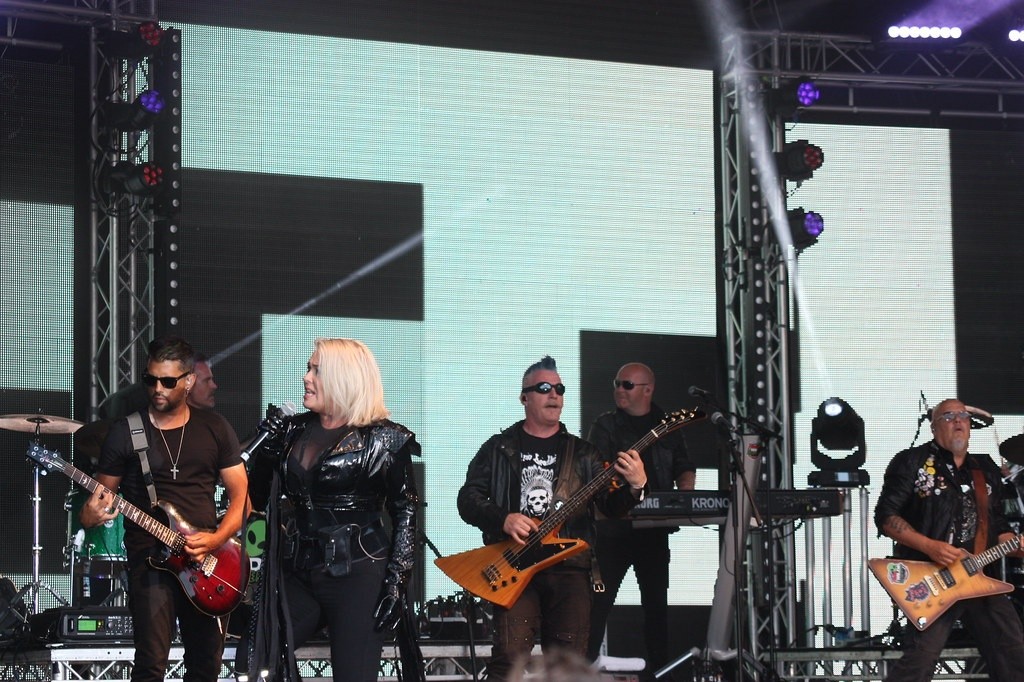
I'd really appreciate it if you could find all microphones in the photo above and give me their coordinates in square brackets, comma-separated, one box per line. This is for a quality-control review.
[240, 402, 296, 462]
[688, 386, 714, 397]
[922, 394, 929, 411]
[712, 411, 740, 434]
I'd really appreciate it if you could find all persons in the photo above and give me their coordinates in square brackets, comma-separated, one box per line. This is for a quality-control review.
[875, 398, 1024, 682]
[585, 363, 696, 682]
[457, 355, 650, 682]
[187, 353, 217, 410]
[80, 337, 248, 682]
[234, 338, 417, 682]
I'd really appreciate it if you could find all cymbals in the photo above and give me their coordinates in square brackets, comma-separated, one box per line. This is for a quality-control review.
[0, 413, 84, 435]
[927, 405, 995, 430]
[999, 433, 1024, 468]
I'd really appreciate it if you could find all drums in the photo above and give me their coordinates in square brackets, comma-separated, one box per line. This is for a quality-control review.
[63, 488, 129, 580]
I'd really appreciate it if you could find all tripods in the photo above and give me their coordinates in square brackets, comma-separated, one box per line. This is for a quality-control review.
[0, 421, 70, 629]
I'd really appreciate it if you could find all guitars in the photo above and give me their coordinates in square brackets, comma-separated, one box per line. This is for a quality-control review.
[867, 530, 1023, 633]
[432, 403, 709, 611]
[25, 439, 252, 620]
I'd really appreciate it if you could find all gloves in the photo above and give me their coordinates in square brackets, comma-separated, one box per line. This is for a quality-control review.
[373, 584, 409, 633]
[258, 408, 286, 448]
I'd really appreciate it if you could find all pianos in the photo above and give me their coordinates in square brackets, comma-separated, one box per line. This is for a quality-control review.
[592, 486, 845, 682]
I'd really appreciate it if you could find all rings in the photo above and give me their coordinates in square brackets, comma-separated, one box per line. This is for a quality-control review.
[105, 507, 110, 513]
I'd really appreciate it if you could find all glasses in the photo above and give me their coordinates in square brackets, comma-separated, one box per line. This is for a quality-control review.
[141, 370, 191, 389]
[932, 412, 970, 427]
[522, 382, 566, 396]
[613, 379, 648, 390]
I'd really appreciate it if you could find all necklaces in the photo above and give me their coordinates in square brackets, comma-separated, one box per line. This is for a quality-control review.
[154, 405, 186, 480]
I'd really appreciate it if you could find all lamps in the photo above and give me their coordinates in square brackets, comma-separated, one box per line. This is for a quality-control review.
[770, 76, 819, 118]
[125, 21, 165, 59]
[123, 163, 165, 197]
[128, 90, 164, 128]
[788, 206, 823, 254]
[871, 23, 966, 53]
[780, 137, 822, 185]
[808, 397, 871, 487]
